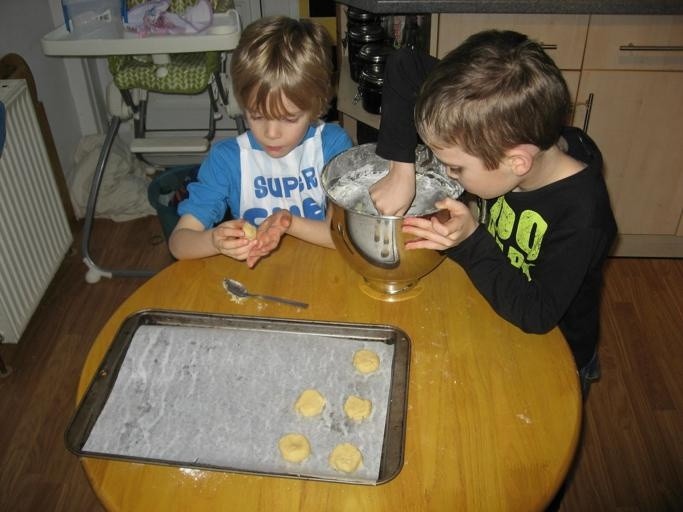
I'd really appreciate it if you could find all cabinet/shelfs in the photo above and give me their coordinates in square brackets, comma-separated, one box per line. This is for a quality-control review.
[333, 0, 683, 261]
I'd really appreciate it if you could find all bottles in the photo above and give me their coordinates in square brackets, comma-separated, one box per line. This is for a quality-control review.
[347, 7, 426, 114]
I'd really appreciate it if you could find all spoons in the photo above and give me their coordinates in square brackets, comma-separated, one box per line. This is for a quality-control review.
[222, 278, 309, 310]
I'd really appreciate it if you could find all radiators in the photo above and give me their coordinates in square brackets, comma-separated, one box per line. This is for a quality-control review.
[0, 79, 74, 378]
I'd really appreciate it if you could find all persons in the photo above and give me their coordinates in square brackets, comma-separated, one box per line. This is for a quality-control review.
[368, 28, 618, 406]
[168, 15, 357, 269]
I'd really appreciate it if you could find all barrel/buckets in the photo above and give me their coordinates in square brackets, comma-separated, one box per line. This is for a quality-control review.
[320, 141, 466, 301]
